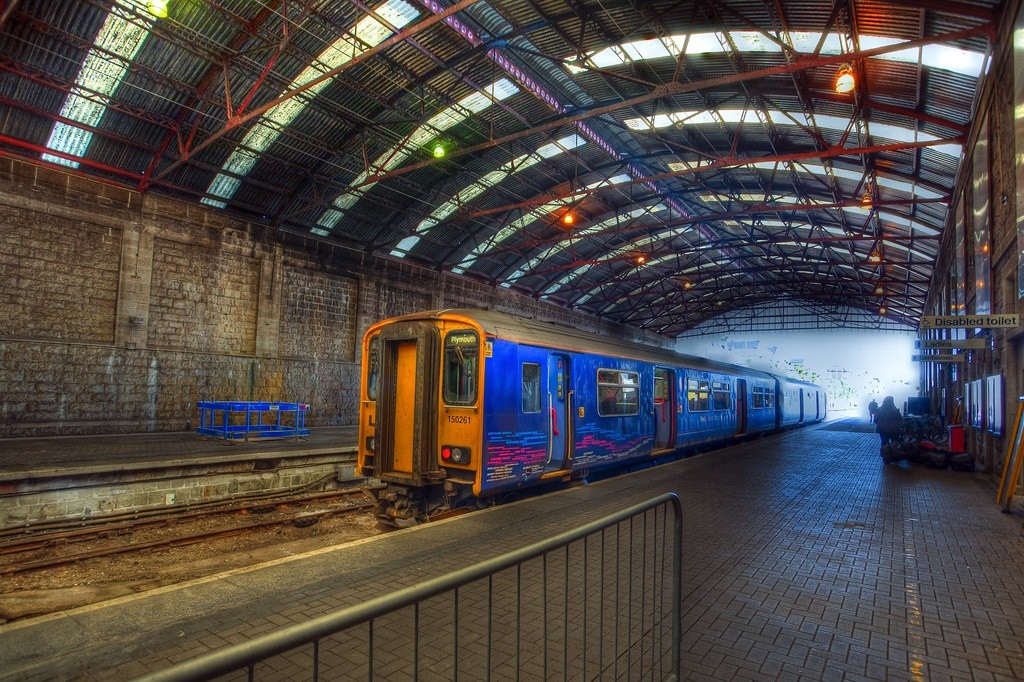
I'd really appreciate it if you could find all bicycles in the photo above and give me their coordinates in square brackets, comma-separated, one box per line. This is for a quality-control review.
[900, 413, 950, 444]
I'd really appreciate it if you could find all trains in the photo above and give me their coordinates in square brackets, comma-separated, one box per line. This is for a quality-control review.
[355, 310, 828, 527]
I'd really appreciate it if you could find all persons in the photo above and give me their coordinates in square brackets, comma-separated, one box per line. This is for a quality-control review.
[874, 396, 904, 445]
[868, 399, 878, 423]
